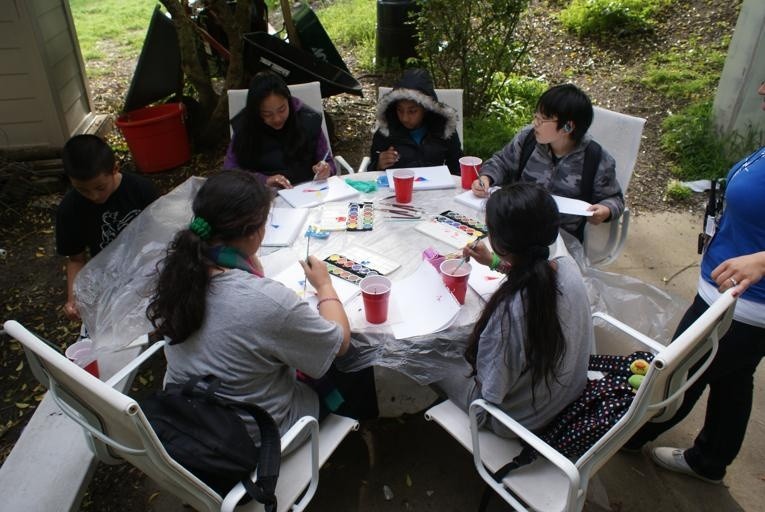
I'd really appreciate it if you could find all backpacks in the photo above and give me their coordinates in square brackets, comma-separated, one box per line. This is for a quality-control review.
[133, 373, 281, 512]
[477, 351, 654, 512]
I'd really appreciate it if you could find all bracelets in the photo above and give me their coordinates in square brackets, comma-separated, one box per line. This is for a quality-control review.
[316, 297, 342, 310]
[490, 252, 500, 271]
[501, 259, 511, 276]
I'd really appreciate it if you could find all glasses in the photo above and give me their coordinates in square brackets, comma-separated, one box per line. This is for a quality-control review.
[534, 115, 558, 126]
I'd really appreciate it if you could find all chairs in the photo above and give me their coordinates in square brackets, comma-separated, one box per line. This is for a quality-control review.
[358, 86, 464, 172]
[227, 80, 355, 175]
[4, 319, 359, 512]
[584, 106, 647, 268]
[424, 286, 742, 512]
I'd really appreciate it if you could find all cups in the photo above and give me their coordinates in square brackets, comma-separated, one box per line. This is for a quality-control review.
[359, 276, 391, 324]
[460, 156, 482, 189]
[440, 259, 471, 305]
[393, 171, 413, 202]
[66, 340, 99, 378]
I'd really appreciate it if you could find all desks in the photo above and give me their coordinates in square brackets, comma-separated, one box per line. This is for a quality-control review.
[255, 171, 566, 347]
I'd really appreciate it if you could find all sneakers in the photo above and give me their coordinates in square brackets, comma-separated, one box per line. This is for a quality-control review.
[621, 446, 640, 453]
[651, 446, 723, 486]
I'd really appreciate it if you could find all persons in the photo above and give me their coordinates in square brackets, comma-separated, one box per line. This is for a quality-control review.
[473, 83, 627, 235]
[424, 182, 593, 441]
[628, 72, 764, 485]
[223, 71, 335, 188]
[145, 173, 391, 512]
[369, 64, 464, 175]
[55, 134, 158, 318]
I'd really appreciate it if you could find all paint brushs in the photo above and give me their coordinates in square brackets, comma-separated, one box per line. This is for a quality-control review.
[384, 216, 420, 219]
[376, 151, 380, 153]
[313, 150, 329, 181]
[304, 236, 309, 286]
[474, 165, 483, 187]
[452, 238, 481, 274]
[384, 210, 408, 213]
[393, 212, 413, 216]
[399, 206, 417, 212]
[386, 203, 414, 208]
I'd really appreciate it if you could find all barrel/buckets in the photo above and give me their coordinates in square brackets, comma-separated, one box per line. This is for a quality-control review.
[114, 103, 192, 173]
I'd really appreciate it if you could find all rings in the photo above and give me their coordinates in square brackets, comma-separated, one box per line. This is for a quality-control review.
[325, 162, 328, 170]
[729, 277, 738, 285]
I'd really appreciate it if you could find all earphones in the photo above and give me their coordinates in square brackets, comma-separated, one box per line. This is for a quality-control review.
[564, 123, 571, 132]
